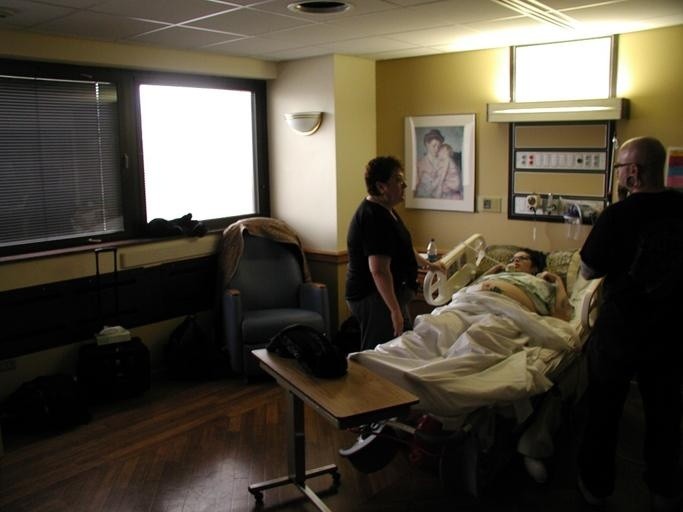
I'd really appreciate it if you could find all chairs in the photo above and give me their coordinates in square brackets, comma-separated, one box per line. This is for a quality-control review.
[216, 216, 331, 378]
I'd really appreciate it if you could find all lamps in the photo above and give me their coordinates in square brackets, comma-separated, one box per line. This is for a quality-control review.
[284, 111, 324, 136]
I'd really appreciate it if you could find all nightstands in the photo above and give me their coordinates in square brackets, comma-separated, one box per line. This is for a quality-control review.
[408, 246, 450, 325]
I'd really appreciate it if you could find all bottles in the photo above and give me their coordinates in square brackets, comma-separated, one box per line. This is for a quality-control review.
[427, 238, 437, 261]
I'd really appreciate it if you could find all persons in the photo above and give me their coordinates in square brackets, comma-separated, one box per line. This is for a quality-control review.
[415, 129, 462, 200]
[344, 157, 448, 351]
[580, 136, 683, 512]
[348, 248, 575, 395]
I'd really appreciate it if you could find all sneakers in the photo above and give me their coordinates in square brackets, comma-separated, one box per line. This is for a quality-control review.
[574, 467, 610, 501]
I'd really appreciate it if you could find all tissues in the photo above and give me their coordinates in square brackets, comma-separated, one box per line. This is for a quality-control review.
[95, 325, 132, 346]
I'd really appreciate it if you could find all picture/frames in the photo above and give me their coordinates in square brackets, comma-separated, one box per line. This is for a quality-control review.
[403, 113, 476, 214]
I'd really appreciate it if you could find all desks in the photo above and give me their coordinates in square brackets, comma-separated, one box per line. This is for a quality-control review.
[248, 346, 421, 512]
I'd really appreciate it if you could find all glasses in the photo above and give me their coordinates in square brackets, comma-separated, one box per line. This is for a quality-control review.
[612, 161, 633, 167]
[511, 255, 533, 261]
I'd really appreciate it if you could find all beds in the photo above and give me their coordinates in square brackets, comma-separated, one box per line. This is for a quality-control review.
[350, 230, 604, 512]
